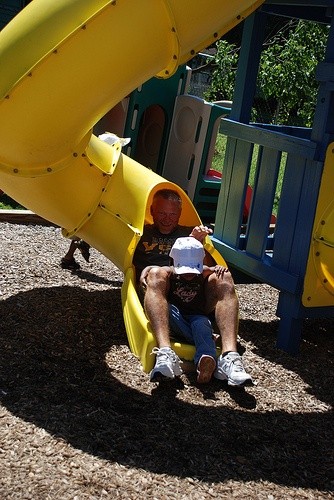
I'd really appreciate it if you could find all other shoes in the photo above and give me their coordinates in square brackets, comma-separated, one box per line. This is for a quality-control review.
[194, 355, 217, 384]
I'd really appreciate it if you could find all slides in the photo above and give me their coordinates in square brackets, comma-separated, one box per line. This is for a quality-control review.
[0, 1, 264, 373]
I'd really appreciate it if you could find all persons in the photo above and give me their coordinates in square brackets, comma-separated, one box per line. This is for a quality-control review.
[139, 236, 230, 384]
[61, 236, 91, 268]
[132, 188, 256, 387]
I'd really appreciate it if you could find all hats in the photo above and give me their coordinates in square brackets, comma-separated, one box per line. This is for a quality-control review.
[169, 236, 207, 277]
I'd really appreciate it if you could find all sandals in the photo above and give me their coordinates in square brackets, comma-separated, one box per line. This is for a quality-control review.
[74, 238, 91, 263]
[60, 255, 82, 269]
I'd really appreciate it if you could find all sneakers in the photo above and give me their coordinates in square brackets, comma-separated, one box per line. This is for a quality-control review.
[212, 353, 254, 389]
[146, 342, 183, 382]
